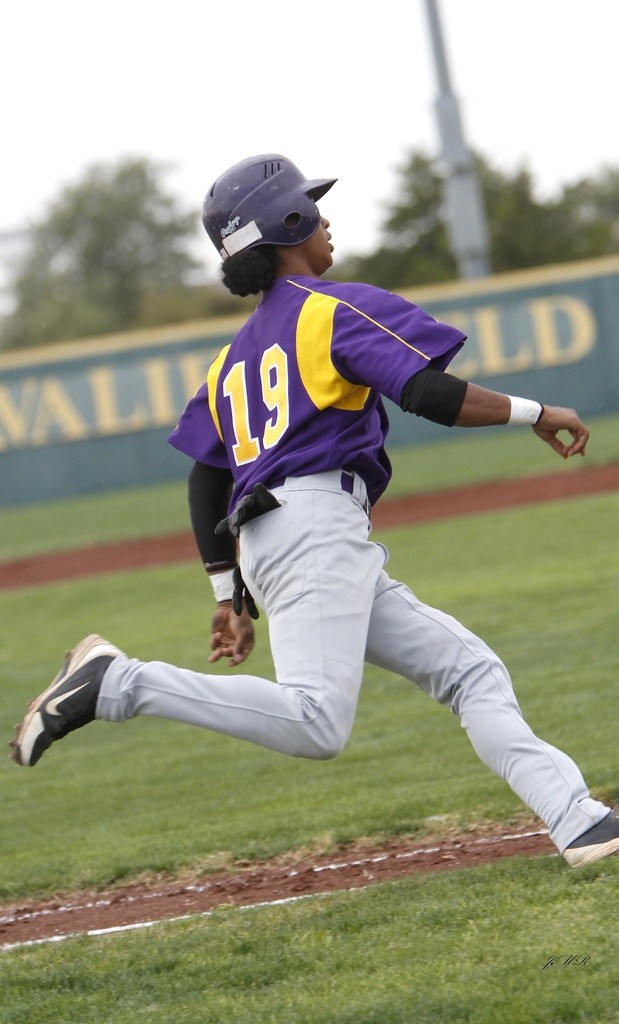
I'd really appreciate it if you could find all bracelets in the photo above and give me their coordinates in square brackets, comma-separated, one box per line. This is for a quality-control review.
[208, 566, 244, 606]
[504, 395, 544, 427]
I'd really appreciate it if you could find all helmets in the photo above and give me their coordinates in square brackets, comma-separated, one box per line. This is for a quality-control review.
[203, 154, 338, 260]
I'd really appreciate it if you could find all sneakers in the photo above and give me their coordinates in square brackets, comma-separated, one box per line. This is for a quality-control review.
[561, 809, 619, 870]
[8, 633, 122, 768]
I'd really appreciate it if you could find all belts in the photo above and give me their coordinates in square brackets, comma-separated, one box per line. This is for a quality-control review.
[268, 472, 373, 522]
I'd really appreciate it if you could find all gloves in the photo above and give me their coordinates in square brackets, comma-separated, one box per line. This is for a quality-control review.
[232, 564, 259, 619]
[214, 482, 281, 537]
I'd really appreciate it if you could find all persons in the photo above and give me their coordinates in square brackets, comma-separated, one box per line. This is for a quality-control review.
[7, 154, 619, 869]
